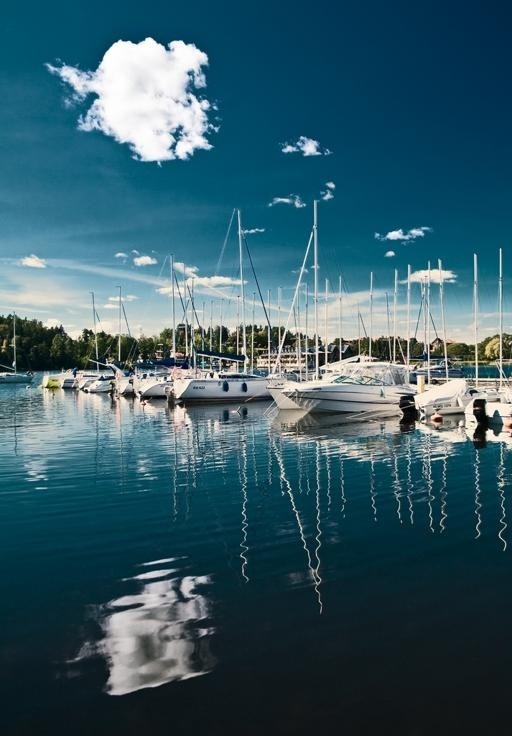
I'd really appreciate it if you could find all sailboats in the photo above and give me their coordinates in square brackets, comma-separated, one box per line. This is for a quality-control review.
[42, 199, 512, 441]
[0, 310, 36, 382]
